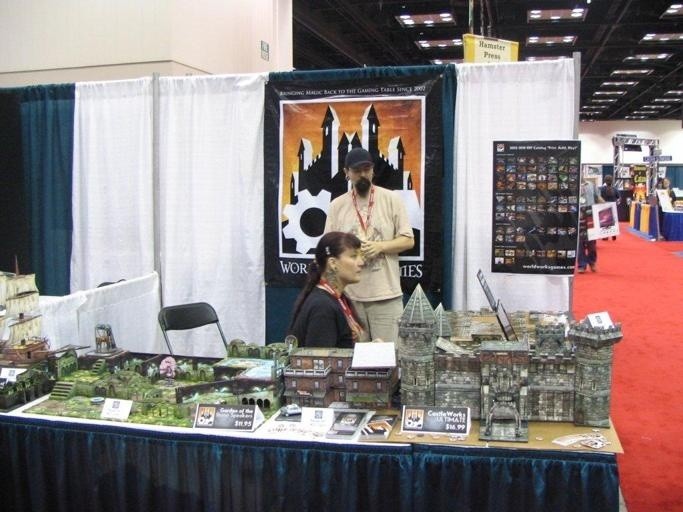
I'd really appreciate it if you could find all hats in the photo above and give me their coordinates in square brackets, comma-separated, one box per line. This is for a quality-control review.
[345, 148, 375, 168]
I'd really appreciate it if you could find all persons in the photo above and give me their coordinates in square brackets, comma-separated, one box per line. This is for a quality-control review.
[656, 177, 665, 190]
[285, 231, 383, 347]
[662, 178, 677, 208]
[595, 175, 619, 242]
[576, 170, 607, 275]
[323, 146, 415, 342]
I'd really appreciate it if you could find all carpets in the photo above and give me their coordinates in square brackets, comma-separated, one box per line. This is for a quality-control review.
[570, 220, 683, 511]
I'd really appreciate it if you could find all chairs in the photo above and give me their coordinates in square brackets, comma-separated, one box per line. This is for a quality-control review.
[158, 300, 230, 361]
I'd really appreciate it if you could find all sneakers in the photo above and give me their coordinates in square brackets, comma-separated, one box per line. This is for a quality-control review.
[579, 269, 584, 273]
[590, 263, 596, 272]
